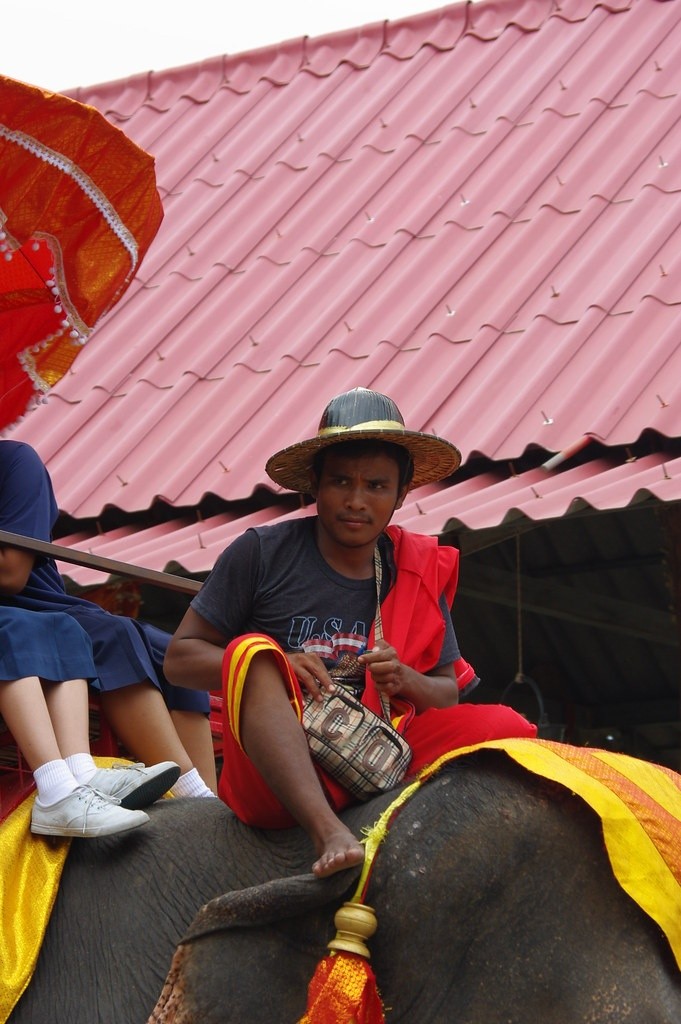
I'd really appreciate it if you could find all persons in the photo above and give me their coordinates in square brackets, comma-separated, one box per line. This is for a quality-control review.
[162, 384, 540, 880]
[0, 439, 219, 839]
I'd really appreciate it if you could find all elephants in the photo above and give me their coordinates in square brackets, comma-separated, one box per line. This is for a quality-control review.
[0, 737, 681, 1024]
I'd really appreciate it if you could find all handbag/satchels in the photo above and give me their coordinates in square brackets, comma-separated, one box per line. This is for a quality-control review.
[302, 680, 413, 802]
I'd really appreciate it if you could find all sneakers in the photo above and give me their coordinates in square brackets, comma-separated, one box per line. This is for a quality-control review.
[85, 761, 181, 810]
[30, 783, 149, 839]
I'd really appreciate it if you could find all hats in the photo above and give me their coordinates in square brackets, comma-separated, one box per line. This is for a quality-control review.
[265, 387, 463, 496]
